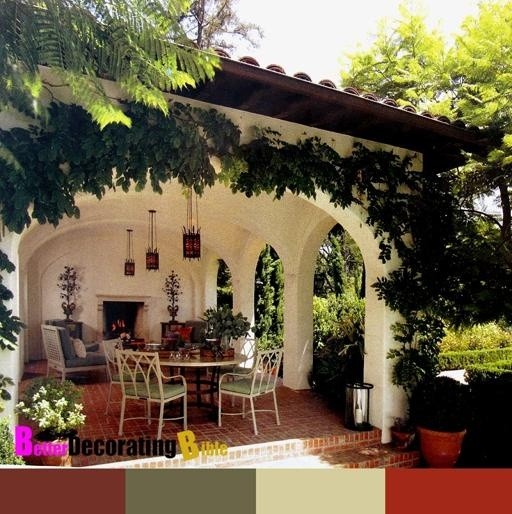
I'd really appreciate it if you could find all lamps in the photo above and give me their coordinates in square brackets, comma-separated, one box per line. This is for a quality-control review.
[182, 185, 200, 261]
[146, 208, 159, 271]
[124, 229, 135, 276]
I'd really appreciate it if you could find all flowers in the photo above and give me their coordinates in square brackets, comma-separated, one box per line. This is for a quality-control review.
[162, 269, 183, 305]
[15, 382, 88, 436]
[56, 265, 80, 305]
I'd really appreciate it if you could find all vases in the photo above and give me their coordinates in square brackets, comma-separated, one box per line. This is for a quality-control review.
[221, 336, 232, 355]
[61, 302, 75, 322]
[33, 431, 70, 465]
[167, 304, 179, 324]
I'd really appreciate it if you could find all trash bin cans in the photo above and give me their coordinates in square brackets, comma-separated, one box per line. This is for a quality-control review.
[345, 381, 373, 432]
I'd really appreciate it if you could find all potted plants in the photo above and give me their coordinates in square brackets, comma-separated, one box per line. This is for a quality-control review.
[411, 377, 477, 468]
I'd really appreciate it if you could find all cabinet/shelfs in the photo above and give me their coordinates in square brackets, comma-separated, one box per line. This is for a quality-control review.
[53, 320, 82, 342]
[161, 322, 185, 344]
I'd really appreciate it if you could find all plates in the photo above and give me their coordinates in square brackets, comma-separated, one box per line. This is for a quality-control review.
[146, 343, 161, 348]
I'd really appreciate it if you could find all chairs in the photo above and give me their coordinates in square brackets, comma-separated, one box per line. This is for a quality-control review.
[218, 348, 282, 436]
[208, 334, 259, 402]
[100, 336, 149, 416]
[116, 350, 188, 442]
[40, 325, 108, 385]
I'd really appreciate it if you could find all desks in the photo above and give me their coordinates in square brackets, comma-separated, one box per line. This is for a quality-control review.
[121, 344, 247, 429]
